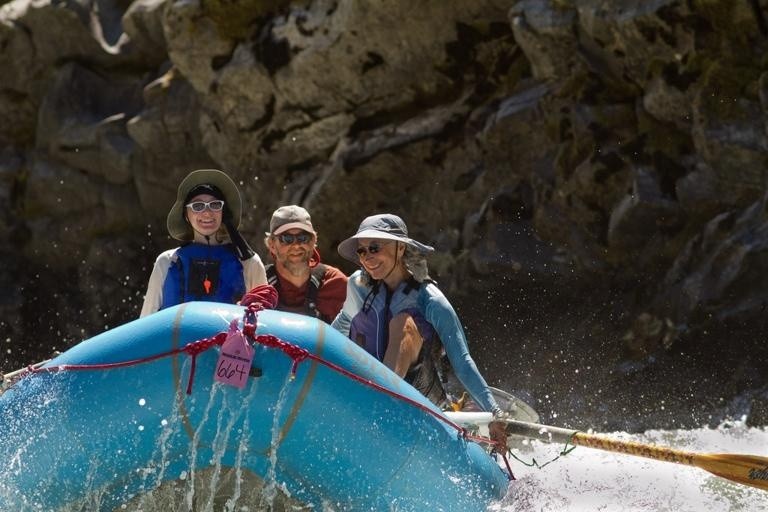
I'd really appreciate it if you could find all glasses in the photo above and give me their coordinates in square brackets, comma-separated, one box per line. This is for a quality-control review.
[357, 242, 390, 256]
[186, 200, 224, 212]
[280, 233, 309, 243]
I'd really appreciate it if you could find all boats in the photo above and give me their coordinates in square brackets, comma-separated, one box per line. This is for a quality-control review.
[1, 299, 509, 512]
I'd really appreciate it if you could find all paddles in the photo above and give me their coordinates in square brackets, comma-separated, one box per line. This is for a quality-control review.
[487, 414, 767, 492]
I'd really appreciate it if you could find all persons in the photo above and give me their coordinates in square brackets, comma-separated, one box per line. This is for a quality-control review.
[329, 213, 508, 431]
[140, 169, 268, 321]
[262, 204, 348, 324]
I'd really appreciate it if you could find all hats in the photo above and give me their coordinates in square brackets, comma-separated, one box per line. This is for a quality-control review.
[337, 214, 435, 266]
[167, 169, 242, 242]
[270, 205, 316, 235]
